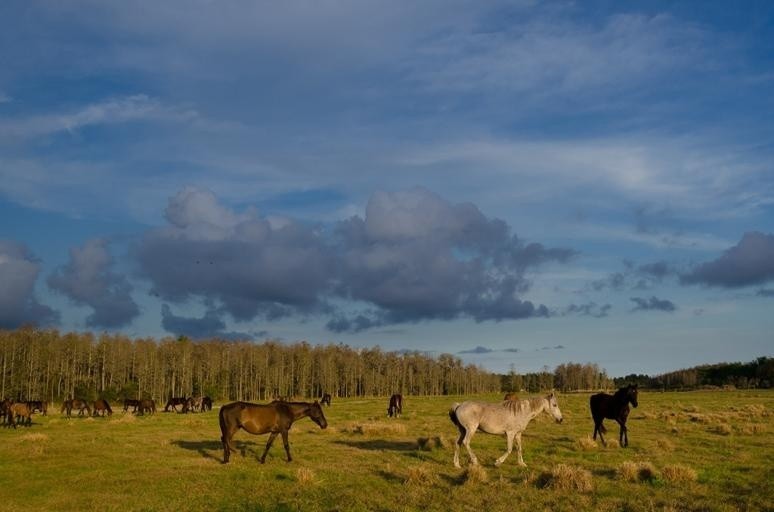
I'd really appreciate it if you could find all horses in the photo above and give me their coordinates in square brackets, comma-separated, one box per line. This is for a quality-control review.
[0, 396, 48, 429]
[387, 393, 403, 418]
[123, 399, 156, 416]
[165, 396, 213, 413]
[61, 399, 92, 420]
[93, 400, 115, 417]
[219, 399, 328, 465]
[589, 382, 639, 447]
[320, 394, 332, 405]
[448, 391, 564, 470]
